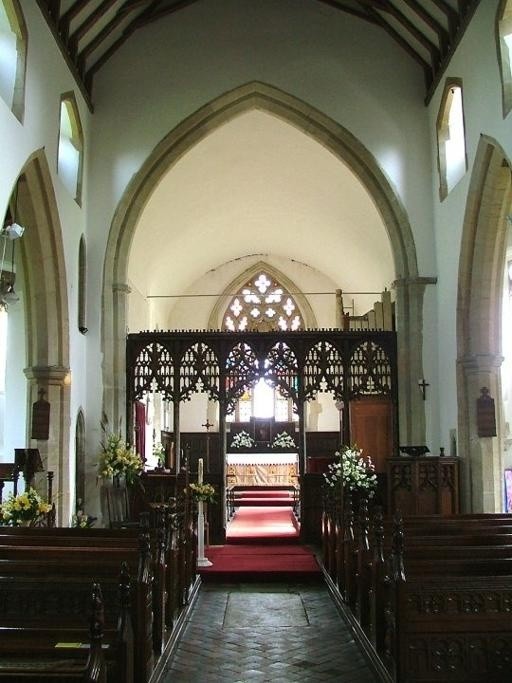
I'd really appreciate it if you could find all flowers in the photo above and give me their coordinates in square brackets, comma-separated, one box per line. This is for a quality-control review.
[91, 409, 148, 495]
[323, 443, 380, 506]
[0, 487, 55, 527]
[182, 480, 220, 503]
[267, 429, 301, 452]
[229, 429, 259, 451]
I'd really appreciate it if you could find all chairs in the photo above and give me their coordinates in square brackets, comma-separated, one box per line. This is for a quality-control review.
[104, 482, 142, 530]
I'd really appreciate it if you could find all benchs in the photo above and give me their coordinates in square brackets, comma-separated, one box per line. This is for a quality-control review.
[336, 513, 512, 681]
[1, 529, 189, 680]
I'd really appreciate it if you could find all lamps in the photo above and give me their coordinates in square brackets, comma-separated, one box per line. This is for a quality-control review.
[0, 219, 27, 317]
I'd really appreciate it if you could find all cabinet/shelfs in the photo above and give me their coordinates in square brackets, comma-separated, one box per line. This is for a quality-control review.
[306, 432, 341, 474]
[384, 456, 462, 516]
[164, 431, 220, 474]
